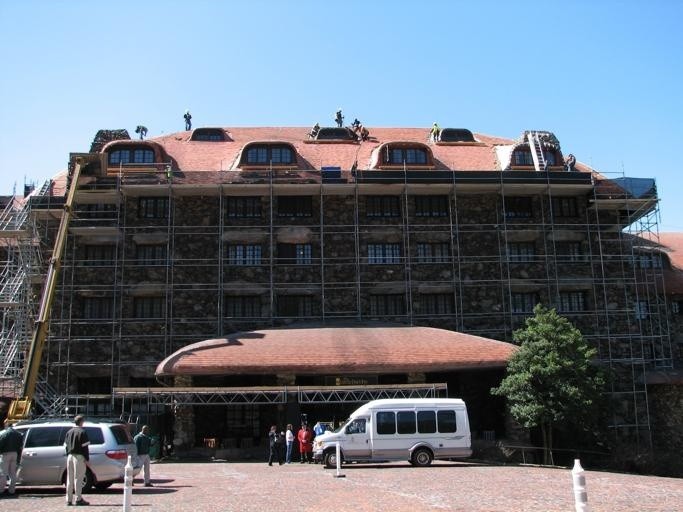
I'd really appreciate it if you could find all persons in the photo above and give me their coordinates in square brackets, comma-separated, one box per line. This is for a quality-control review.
[268, 421, 325, 466]
[184, 110, 192, 131]
[0, 420, 24, 498]
[134, 125, 148, 139]
[565, 153, 576, 172]
[131, 425, 154, 487]
[63, 415, 91, 506]
[429, 121, 440, 141]
[306, 109, 369, 142]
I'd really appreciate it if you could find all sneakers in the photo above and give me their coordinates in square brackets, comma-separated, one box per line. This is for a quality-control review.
[75, 498, 89, 505]
[144, 483, 153, 487]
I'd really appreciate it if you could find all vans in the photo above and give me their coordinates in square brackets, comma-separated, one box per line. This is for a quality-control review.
[0, 420, 140, 493]
[314, 396, 475, 468]
